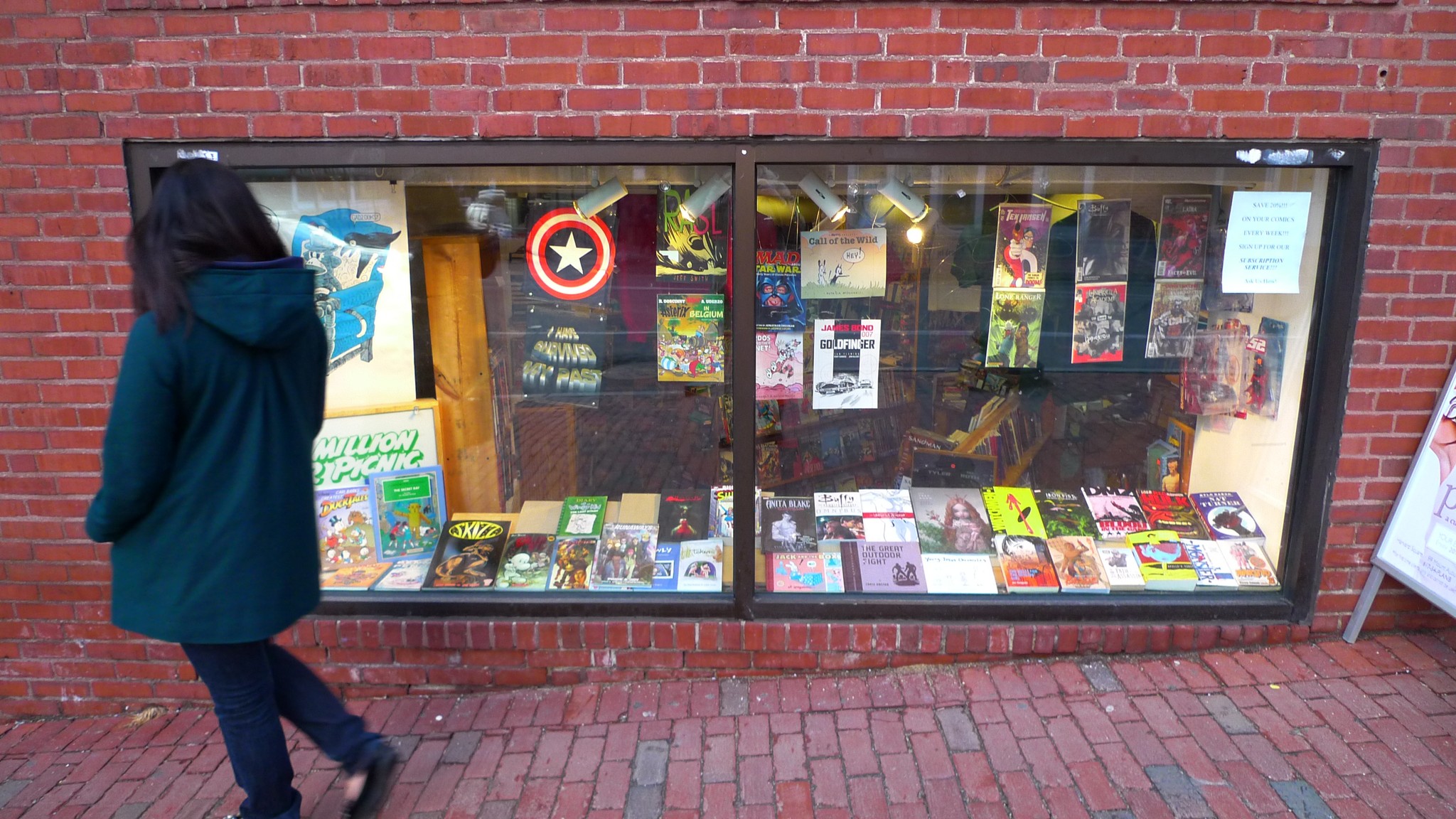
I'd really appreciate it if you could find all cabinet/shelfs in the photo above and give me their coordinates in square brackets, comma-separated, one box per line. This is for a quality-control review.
[485, 186, 924, 507]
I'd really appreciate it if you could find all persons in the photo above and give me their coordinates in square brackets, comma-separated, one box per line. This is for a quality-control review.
[84, 155, 411, 819]
[832, 516, 857, 539]
[770, 510, 801, 553]
[821, 519, 840, 539]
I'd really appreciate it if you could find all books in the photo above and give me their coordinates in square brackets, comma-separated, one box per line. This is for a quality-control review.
[754, 162, 1290, 595]
[312, 185, 733, 592]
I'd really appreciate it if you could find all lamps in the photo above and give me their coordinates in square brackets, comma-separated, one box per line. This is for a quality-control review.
[676, 167, 735, 223]
[796, 167, 851, 224]
[571, 173, 635, 220]
[877, 172, 932, 224]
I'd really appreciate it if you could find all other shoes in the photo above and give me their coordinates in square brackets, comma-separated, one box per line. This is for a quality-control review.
[223, 812, 244, 819]
[342, 746, 396, 819]
